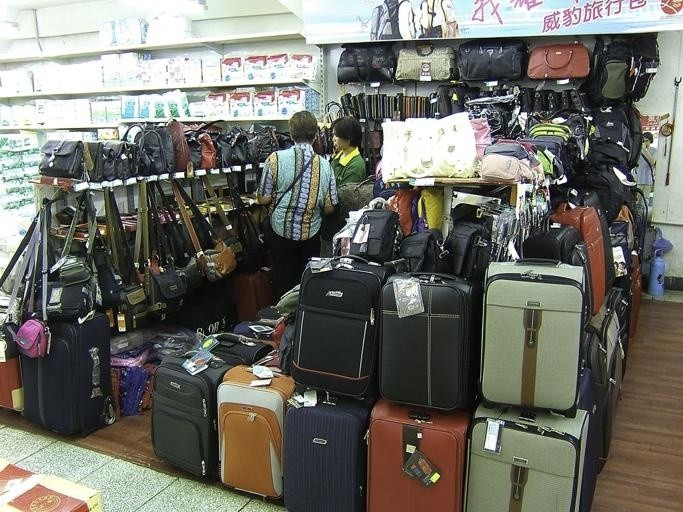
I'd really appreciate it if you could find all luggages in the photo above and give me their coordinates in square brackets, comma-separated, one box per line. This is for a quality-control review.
[0, 316, 288, 508]
[289, 258, 642, 512]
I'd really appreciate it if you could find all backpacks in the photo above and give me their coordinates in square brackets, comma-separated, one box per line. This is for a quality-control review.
[581, 36, 659, 106]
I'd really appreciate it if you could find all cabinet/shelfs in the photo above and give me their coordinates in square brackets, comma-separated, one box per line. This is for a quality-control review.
[0, 34, 331, 315]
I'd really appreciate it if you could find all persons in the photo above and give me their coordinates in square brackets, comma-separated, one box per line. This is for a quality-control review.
[320, 116, 366, 257]
[370, 0, 458, 40]
[636, 131, 657, 216]
[257, 111, 339, 306]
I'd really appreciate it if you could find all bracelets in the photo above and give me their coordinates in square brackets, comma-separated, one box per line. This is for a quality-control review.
[643, 149, 647, 155]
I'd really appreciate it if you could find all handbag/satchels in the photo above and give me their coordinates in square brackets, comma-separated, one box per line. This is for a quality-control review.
[456, 41, 530, 83]
[329, 87, 650, 259]
[525, 45, 590, 79]
[1, 122, 327, 312]
[393, 43, 459, 82]
[335, 46, 397, 85]
